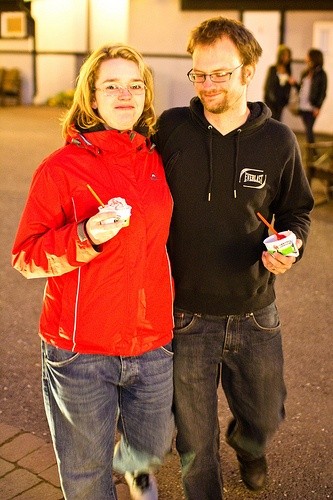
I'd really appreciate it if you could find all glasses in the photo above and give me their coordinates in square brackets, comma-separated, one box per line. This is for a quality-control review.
[92, 84, 147, 95]
[187, 61, 244, 83]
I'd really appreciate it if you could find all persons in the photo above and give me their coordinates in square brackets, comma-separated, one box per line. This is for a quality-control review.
[11, 43, 176, 500]
[263, 45, 327, 175]
[150, 16, 315, 500]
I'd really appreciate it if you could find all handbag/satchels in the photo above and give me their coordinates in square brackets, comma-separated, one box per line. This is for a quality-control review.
[287, 92, 301, 112]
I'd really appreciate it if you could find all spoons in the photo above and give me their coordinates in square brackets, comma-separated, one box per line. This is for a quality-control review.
[256, 212, 287, 241]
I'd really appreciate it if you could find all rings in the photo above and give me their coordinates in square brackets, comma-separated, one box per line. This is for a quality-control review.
[272, 267, 277, 270]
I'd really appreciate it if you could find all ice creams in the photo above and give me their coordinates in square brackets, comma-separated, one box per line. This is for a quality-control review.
[97, 197, 132, 227]
[263, 230, 299, 257]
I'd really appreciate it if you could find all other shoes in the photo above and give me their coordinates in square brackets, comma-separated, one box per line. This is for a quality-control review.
[124, 467, 157, 500]
[238, 454, 268, 491]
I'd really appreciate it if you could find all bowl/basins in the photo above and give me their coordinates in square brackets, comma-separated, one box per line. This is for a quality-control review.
[98, 204, 132, 229]
[263, 230, 300, 259]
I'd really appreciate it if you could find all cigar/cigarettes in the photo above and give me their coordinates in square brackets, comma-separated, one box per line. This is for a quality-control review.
[100, 218, 117, 225]
